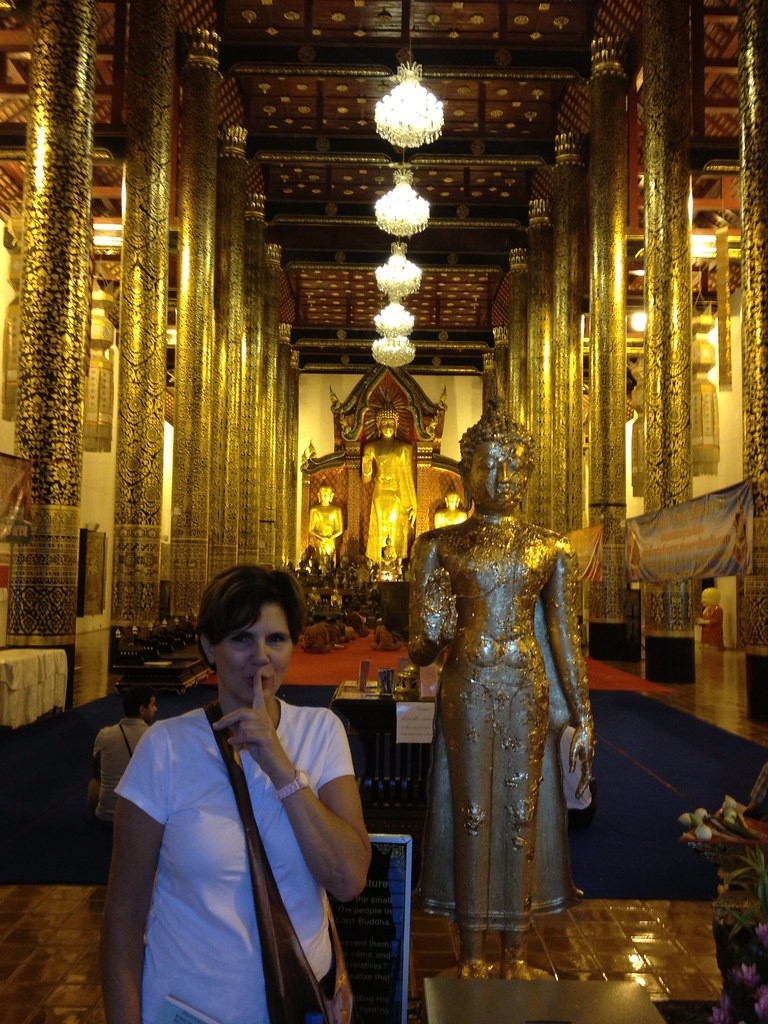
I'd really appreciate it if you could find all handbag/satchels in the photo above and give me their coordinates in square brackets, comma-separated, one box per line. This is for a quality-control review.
[203, 700, 354, 1024]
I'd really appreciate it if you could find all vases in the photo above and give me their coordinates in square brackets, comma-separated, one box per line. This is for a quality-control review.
[711, 901, 768, 1017]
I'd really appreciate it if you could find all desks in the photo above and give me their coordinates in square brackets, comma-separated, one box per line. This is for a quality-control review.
[0, 648, 69, 730]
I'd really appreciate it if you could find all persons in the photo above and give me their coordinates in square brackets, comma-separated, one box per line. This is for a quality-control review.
[92, 687, 160, 828]
[434, 491, 467, 528]
[308, 484, 344, 569]
[362, 405, 418, 563]
[318, 555, 331, 575]
[696, 588, 724, 650]
[99, 564, 372, 1024]
[300, 604, 401, 653]
[381, 538, 401, 568]
[406, 398, 595, 979]
[288, 561, 318, 584]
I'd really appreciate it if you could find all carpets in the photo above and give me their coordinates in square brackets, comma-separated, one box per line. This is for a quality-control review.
[0, 683, 768, 901]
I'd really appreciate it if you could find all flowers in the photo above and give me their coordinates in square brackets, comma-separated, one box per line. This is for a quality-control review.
[678, 762, 768, 1024]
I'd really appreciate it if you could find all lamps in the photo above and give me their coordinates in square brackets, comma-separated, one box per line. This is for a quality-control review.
[374, 148, 430, 237]
[372, 236, 422, 368]
[374, 0, 445, 148]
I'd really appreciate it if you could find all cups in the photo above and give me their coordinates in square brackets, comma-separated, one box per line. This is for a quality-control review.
[378, 668, 394, 696]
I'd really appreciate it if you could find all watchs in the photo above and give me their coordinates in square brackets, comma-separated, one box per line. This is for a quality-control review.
[276, 772, 310, 800]
